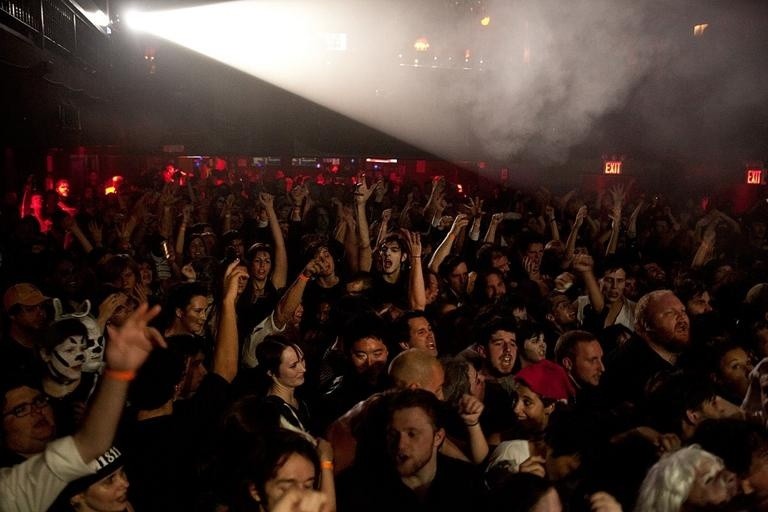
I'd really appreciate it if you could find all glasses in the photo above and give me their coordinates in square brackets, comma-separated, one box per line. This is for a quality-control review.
[2, 392, 54, 418]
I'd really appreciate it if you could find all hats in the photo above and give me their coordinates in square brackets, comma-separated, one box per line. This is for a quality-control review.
[513, 360, 578, 406]
[65, 440, 127, 501]
[2, 283, 52, 316]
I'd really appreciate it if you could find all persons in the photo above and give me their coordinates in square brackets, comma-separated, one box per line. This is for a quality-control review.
[3, 159, 766, 512]
